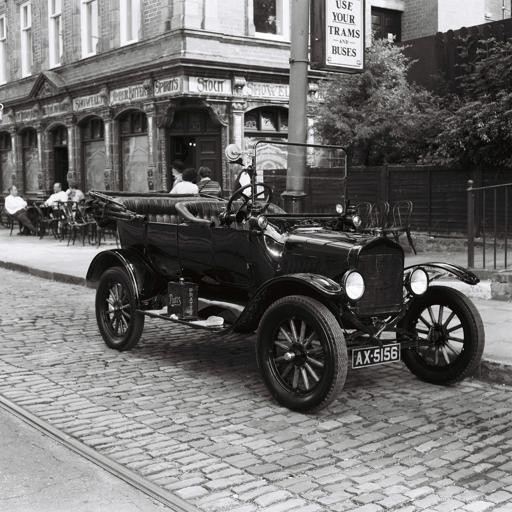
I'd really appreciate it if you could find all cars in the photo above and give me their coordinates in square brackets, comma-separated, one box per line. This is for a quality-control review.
[81, 138, 486, 417]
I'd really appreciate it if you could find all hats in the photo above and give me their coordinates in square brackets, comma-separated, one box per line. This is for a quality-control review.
[183, 168, 197, 181]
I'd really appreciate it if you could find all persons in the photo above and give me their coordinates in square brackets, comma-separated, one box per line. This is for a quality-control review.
[227, 157, 252, 202]
[3, 185, 47, 238]
[168, 159, 185, 185]
[167, 168, 200, 195]
[195, 166, 224, 200]
[40, 182, 69, 209]
[58, 183, 85, 237]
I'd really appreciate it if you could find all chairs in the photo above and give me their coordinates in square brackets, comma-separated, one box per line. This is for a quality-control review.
[350, 199, 417, 257]
[0, 198, 119, 249]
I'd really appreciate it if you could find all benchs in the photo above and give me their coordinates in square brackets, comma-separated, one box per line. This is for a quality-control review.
[115, 189, 288, 239]
